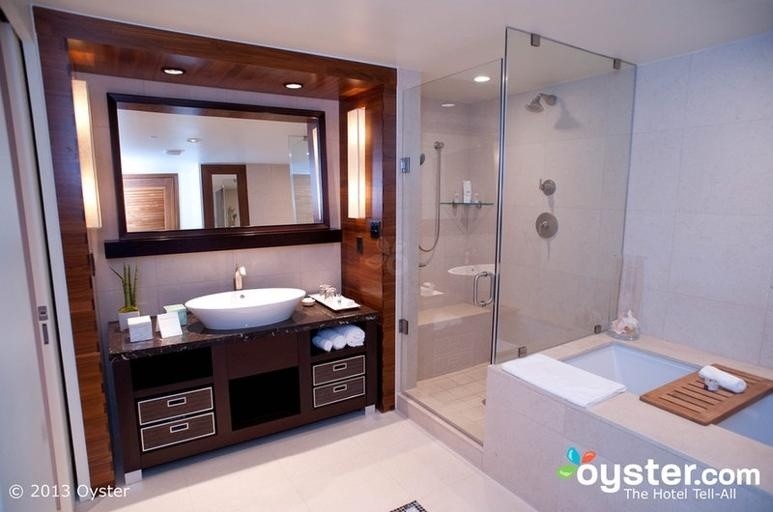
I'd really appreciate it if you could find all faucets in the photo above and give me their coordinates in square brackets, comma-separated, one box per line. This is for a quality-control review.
[234, 265, 246, 290]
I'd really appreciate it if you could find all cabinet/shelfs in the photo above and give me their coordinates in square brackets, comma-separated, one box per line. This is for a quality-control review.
[112, 321, 378, 484]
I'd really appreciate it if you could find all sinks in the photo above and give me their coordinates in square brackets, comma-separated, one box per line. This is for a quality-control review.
[448, 264, 494, 290]
[184, 287, 306, 331]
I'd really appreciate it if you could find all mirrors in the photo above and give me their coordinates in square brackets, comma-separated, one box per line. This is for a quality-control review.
[105, 89, 331, 239]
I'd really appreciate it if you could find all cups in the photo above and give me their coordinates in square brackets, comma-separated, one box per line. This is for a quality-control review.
[319, 283, 337, 306]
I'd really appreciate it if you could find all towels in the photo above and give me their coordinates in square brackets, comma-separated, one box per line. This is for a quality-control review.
[699, 363, 747, 394]
[499, 349, 624, 407]
[311, 324, 365, 354]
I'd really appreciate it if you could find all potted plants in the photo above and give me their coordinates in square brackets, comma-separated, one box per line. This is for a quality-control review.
[110, 262, 141, 331]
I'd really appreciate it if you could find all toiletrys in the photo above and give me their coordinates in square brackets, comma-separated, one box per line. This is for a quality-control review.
[462, 180, 472, 203]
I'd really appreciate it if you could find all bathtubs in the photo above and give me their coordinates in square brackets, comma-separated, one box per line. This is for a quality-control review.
[555, 342, 772, 448]
